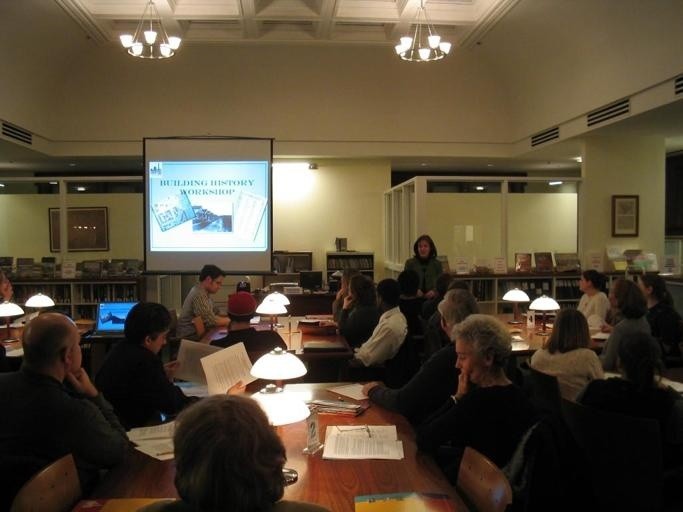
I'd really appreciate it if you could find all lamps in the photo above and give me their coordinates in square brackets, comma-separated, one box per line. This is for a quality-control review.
[0, 292, 55, 343]
[392, 2, 451, 64]
[253, 291, 290, 330]
[501, 287, 560, 336]
[119, 1, 181, 60]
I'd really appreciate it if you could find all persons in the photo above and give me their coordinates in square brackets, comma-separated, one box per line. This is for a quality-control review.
[0, 312, 130, 512]
[89, 301, 246, 428]
[142, 394, 327, 511]
[363, 288, 540, 483]
[529, 270, 683, 402]
[175, 264, 231, 339]
[209, 292, 288, 352]
[332, 268, 470, 381]
[572, 329, 682, 471]
[403, 234, 443, 297]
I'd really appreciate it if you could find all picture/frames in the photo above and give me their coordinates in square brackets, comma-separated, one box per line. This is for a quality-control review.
[47, 205, 108, 252]
[610, 193, 640, 236]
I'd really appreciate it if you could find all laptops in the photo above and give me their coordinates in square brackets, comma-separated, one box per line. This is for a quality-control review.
[91, 301, 140, 339]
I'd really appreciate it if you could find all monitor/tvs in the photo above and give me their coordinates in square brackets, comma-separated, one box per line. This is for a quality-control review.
[272, 252, 313, 273]
[300, 271, 323, 289]
[42, 256, 55, 262]
[17, 258, 34, 266]
[0, 255, 14, 271]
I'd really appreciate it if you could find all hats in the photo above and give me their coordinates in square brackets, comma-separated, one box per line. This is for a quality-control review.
[227, 291, 256, 321]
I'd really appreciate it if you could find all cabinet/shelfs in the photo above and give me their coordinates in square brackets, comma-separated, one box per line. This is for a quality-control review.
[0, 277, 140, 329]
[443, 270, 657, 313]
[263, 250, 376, 286]
